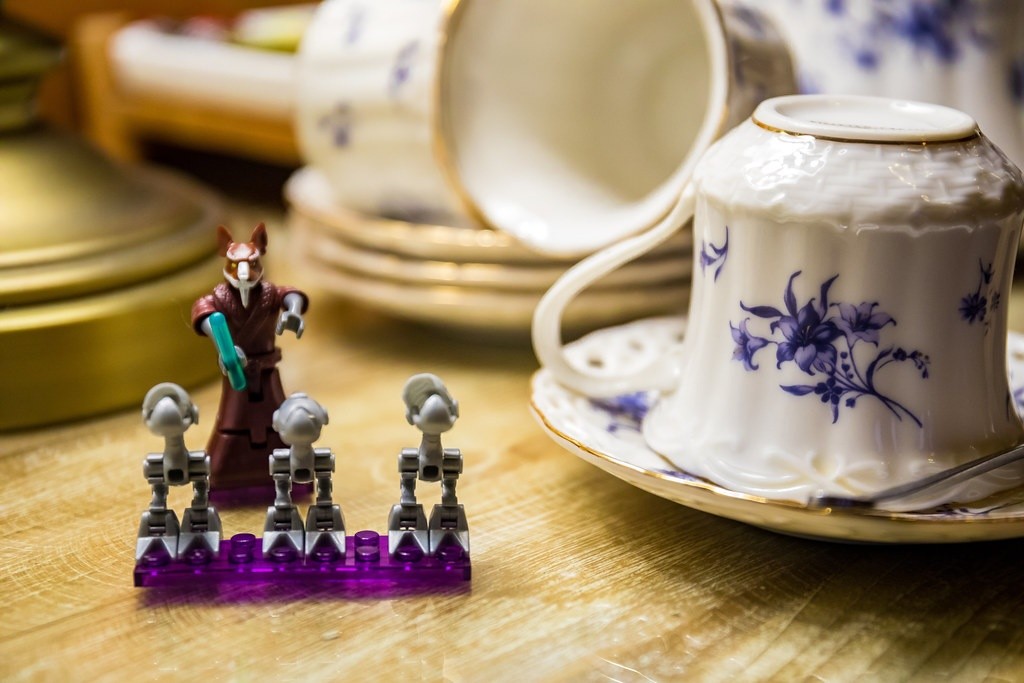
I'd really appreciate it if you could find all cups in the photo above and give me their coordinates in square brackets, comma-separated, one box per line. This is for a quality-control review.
[532, 94, 1024, 513]
[729, 0, 1024, 175]
[290, 0, 801, 261]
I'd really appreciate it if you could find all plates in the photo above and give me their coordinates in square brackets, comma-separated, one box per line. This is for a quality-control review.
[527, 313, 1024, 541]
[282, 171, 691, 339]
